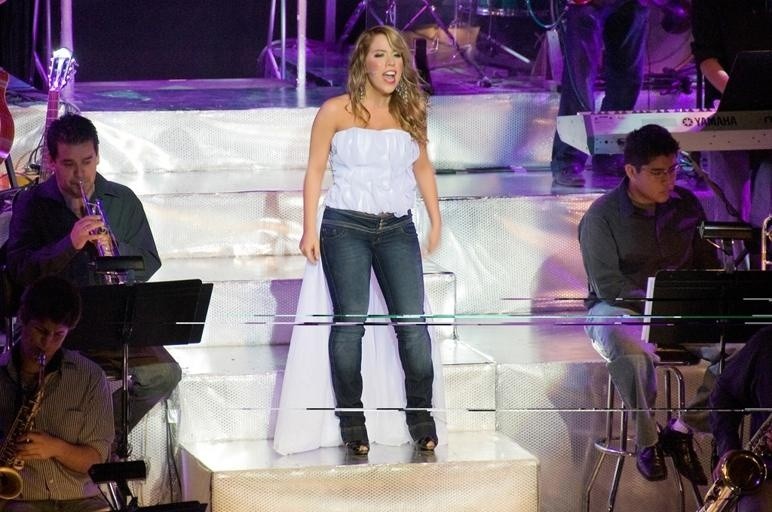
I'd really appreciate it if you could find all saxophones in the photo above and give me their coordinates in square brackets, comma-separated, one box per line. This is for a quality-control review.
[0, 347, 46, 501]
[694, 411, 772, 512]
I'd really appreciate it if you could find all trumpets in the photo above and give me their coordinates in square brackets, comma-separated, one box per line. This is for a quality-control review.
[76, 180, 132, 287]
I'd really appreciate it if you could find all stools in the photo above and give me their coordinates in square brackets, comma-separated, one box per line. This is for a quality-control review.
[583, 349, 710, 511]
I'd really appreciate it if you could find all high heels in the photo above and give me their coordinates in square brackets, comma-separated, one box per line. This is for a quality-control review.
[416, 437, 439, 449]
[346, 441, 369, 457]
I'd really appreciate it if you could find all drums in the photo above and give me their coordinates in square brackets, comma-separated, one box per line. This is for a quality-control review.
[459, 0, 552, 18]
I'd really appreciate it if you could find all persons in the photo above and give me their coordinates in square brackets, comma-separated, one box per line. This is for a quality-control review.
[577, 125, 746, 486]
[550, 0, 651, 186]
[0, 110, 182, 464]
[0, 275, 115, 512]
[300, 25, 442, 454]
[687, 0, 772, 270]
[710, 325, 772, 512]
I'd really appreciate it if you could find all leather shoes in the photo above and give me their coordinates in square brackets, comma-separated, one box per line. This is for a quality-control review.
[634, 419, 708, 486]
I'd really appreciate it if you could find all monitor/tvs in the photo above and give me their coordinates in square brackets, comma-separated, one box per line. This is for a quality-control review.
[717, 49, 772, 113]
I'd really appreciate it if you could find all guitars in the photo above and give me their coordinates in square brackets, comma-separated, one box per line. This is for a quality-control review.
[38, 47, 80, 185]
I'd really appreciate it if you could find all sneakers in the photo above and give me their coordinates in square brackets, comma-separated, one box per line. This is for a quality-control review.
[552, 165, 586, 186]
[592, 154, 626, 176]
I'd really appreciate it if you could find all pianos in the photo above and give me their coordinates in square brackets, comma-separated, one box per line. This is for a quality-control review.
[556, 109, 772, 157]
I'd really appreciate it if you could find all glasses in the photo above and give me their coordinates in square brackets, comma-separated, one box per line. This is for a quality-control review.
[631, 165, 681, 177]
